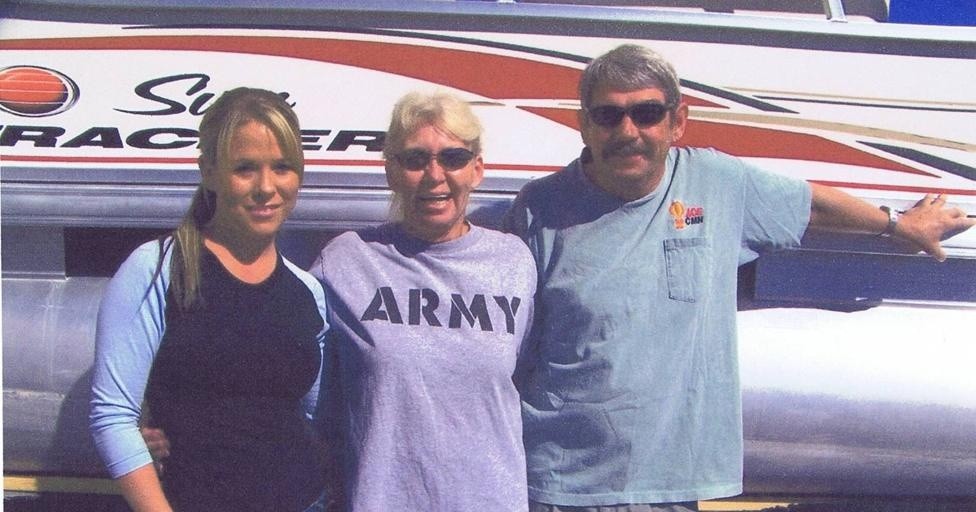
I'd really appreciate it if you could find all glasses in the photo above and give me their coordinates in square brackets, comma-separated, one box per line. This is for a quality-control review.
[584, 99, 674, 127]
[394, 147, 477, 172]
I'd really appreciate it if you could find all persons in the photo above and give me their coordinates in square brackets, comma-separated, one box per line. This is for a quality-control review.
[136, 88, 539, 511]
[87, 86, 337, 510]
[493, 42, 975, 509]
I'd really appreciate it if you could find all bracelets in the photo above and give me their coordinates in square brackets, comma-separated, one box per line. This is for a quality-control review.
[870, 204, 901, 241]
